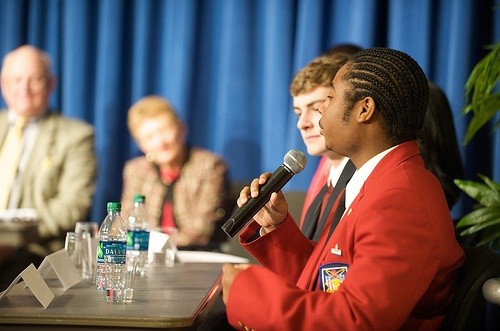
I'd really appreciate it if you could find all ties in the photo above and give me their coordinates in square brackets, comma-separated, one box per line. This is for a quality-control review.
[0, 117, 27, 209]
[318, 182, 335, 225]
[327, 190, 346, 241]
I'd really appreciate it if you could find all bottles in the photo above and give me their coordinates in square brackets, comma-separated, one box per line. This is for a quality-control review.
[125, 195, 151, 278]
[95, 203, 128, 294]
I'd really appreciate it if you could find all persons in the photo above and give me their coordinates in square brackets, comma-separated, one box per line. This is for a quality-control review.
[221, 45, 468, 331]
[0, 44, 98, 263]
[289, 41, 462, 246]
[112, 95, 231, 257]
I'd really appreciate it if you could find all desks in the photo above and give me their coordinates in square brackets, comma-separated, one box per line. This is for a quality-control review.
[0, 261, 225, 331]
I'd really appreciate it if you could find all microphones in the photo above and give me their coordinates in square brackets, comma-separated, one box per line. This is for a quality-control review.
[221, 150, 307, 238]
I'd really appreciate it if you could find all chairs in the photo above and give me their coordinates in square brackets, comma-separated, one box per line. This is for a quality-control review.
[442, 247, 500, 331]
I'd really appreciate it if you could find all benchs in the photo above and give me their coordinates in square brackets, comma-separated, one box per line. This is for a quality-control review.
[187, 190, 305, 264]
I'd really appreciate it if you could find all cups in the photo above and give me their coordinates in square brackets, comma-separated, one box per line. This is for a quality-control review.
[74, 221, 98, 285]
[64, 231, 93, 279]
[153, 227, 175, 267]
[104, 254, 140, 304]
